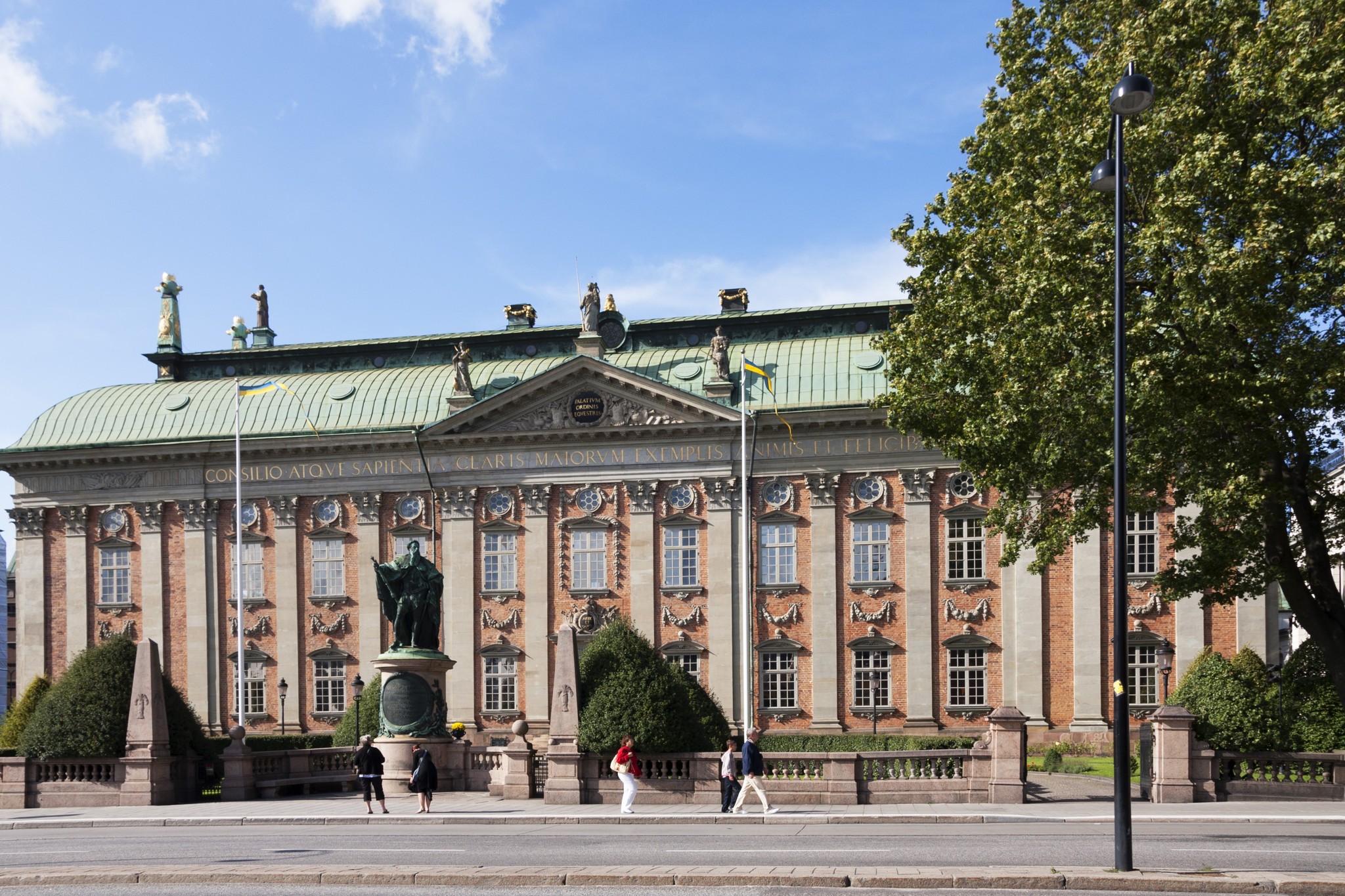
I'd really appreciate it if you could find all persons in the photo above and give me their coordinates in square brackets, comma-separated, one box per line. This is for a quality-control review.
[592, 282, 600, 300]
[720, 739, 742, 813]
[710, 326, 729, 377]
[409, 743, 436, 814]
[452, 341, 473, 391]
[617, 733, 642, 813]
[373, 540, 439, 649]
[251, 285, 269, 327]
[733, 727, 780, 814]
[352, 734, 389, 814]
[580, 282, 599, 332]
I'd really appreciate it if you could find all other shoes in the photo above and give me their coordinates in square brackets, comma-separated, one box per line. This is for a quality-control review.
[722, 806, 733, 813]
[621, 808, 634, 813]
[414, 808, 429, 813]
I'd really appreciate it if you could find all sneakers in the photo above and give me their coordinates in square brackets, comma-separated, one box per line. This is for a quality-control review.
[764, 807, 779, 814]
[733, 809, 748, 814]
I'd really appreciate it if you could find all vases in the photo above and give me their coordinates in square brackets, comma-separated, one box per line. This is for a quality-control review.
[451, 729, 466, 740]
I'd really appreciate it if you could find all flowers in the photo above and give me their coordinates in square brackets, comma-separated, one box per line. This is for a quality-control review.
[452, 723, 465, 730]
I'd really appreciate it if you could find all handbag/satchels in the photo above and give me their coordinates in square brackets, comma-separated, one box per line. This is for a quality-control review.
[610, 747, 631, 774]
[412, 767, 419, 783]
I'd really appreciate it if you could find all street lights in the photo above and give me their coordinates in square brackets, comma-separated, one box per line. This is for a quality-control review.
[1154, 637, 1176, 705]
[276, 677, 288, 734]
[350, 672, 365, 747]
[868, 669, 880, 734]
[1090, 59, 1156, 872]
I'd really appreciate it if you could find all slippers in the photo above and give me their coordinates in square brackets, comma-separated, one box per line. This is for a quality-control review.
[367, 810, 373, 814]
[382, 809, 389, 813]
